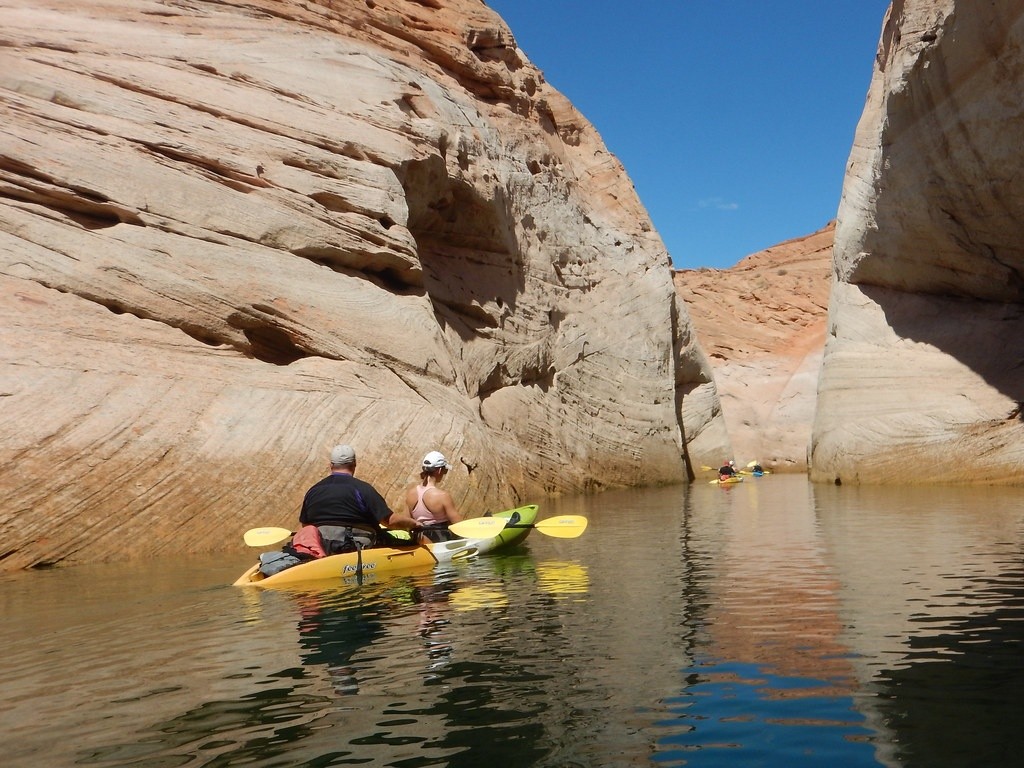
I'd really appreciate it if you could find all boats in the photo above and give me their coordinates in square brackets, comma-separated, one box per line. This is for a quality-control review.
[737, 468, 771, 477]
[707, 476, 745, 487]
[230, 502, 540, 594]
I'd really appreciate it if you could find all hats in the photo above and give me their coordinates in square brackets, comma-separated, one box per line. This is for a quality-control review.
[330, 445, 356, 464]
[423, 450, 454, 470]
[729, 461, 734, 465]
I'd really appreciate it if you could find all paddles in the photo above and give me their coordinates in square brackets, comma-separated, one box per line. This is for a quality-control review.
[385, 513, 589, 541]
[242, 516, 510, 548]
[700, 465, 770, 476]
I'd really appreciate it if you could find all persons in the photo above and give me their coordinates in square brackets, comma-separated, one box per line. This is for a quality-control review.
[717, 459, 735, 481]
[752, 463, 763, 472]
[406, 451, 521, 546]
[298, 445, 424, 555]
